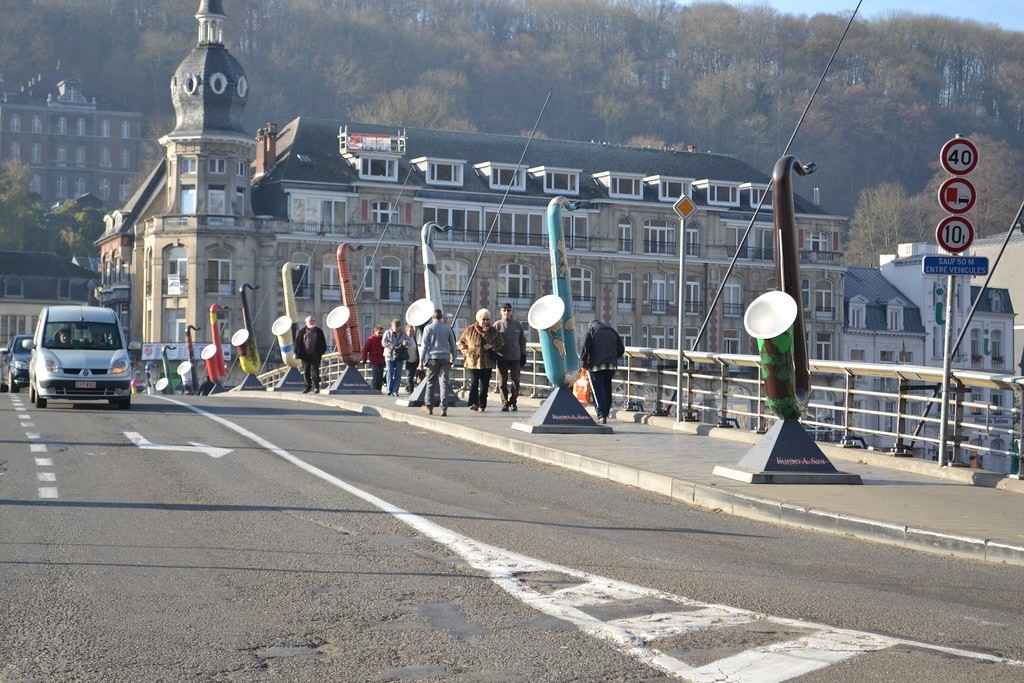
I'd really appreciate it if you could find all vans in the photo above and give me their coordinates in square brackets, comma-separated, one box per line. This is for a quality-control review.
[20, 304, 142, 409]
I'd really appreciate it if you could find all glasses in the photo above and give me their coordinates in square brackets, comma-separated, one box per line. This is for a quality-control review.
[376, 331, 383, 334]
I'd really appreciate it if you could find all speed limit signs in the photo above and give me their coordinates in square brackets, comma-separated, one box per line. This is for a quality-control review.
[941, 139, 978, 175]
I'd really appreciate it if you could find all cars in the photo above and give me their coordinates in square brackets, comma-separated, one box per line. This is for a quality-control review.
[0, 334, 38, 394]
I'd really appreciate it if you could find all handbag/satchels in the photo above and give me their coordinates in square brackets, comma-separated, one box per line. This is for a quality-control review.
[573, 370, 590, 405]
[392, 343, 410, 362]
[484, 341, 504, 360]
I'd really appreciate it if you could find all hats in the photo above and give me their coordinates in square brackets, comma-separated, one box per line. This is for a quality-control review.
[503, 303, 512, 308]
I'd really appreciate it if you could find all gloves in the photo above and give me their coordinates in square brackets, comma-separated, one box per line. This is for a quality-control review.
[363, 359, 367, 365]
[521, 354, 526, 367]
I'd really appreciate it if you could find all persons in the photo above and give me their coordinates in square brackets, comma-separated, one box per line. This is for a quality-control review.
[458, 308, 505, 411]
[57, 329, 71, 345]
[381, 319, 412, 396]
[89, 332, 106, 347]
[293, 315, 327, 394]
[361, 325, 386, 390]
[404, 325, 419, 394]
[420, 309, 457, 416]
[493, 303, 527, 411]
[580, 320, 624, 423]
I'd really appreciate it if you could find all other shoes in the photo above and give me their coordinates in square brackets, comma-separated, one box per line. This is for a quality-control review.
[303, 387, 310, 394]
[440, 409, 446, 417]
[478, 407, 484, 412]
[511, 403, 517, 412]
[594, 418, 606, 424]
[470, 404, 478, 412]
[421, 404, 432, 415]
[315, 389, 319, 393]
[501, 404, 509, 411]
